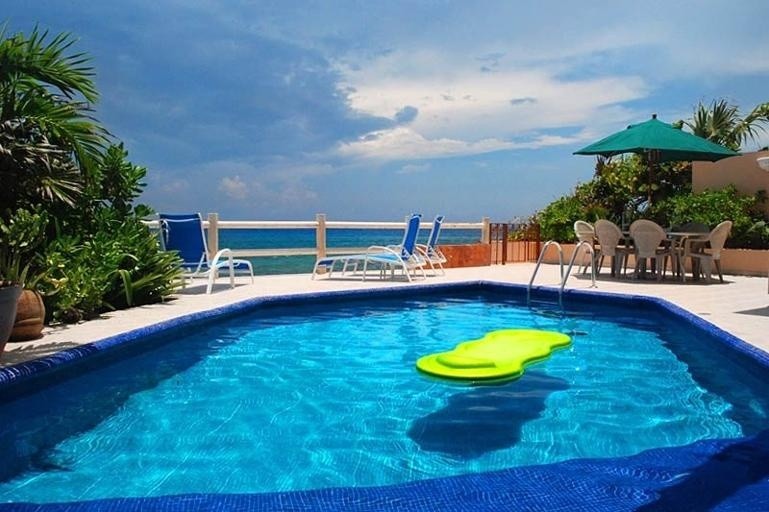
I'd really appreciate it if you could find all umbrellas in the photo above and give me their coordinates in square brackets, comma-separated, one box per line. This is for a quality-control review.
[572, 114, 742, 219]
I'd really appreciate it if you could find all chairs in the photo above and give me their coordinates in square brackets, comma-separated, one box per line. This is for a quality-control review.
[572, 218, 732, 283]
[157, 212, 255, 289]
[310, 213, 447, 282]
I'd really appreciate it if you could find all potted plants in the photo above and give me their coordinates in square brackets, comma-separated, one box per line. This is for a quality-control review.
[0, 191, 70, 353]
